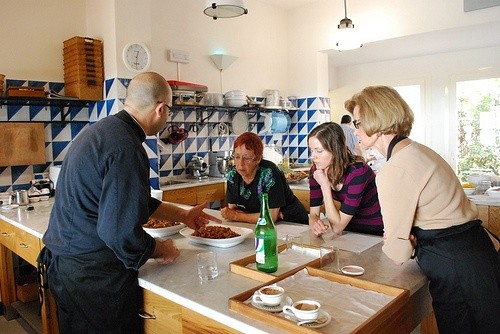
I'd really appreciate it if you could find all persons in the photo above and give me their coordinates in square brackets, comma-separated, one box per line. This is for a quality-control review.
[221, 132, 308, 225]
[344, 86, 500, 334]
[35, 72, 222, 334]
[307, 122, 384, 237]
[340, 114, 377, 164]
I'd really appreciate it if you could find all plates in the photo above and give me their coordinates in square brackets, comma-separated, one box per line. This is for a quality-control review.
[342, 266, 365, 275]
[29, 197, 40, 202]
[2, 205, 19, 210]
[284, 308, 331, 329]
[251, 294, 293, 312]
[40, 196, 49, 200]
[232, 112, 249, 134]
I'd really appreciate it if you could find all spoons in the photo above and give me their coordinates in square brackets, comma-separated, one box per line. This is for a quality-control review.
[296, 318, 327, 327]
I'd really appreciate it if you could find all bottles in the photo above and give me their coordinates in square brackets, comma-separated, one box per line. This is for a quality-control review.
[254, 193, 278, 273]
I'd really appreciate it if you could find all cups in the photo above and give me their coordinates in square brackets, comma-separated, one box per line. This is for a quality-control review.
[320, 246, 340, 273]
[280, 100, 286, 107]
[283, 299, 319, 324]
[12, 190, 28, 206]
[252, 286, 285, 307]
[197, 252, 219, 280]
[286, 234, 303, 256]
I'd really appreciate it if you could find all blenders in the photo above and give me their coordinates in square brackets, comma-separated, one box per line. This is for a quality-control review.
[207, 151, 231, 178]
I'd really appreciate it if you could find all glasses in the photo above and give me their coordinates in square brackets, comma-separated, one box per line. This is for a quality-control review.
[353, 120, 361, 129]
[157, 101, 175, 116]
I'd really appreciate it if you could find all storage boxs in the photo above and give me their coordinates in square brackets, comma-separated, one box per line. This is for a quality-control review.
[62, 36, 104, 100]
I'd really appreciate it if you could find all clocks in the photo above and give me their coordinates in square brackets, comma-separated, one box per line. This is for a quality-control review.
[122, 41, 152, 72]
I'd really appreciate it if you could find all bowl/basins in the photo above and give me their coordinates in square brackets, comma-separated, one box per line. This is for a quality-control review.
[223, 89, 246, 107]
[217, 160, 232, 174]
[143, 224, 186, 238]
[462, 187, 476, 195]
[178, 224, 253, 248]
[487, 187, 500, 199]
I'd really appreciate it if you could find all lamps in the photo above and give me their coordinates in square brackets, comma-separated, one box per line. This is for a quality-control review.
[210, 54, 234, 69]
[334, 0, 363, 51]
[203, 0, 248, 21]
